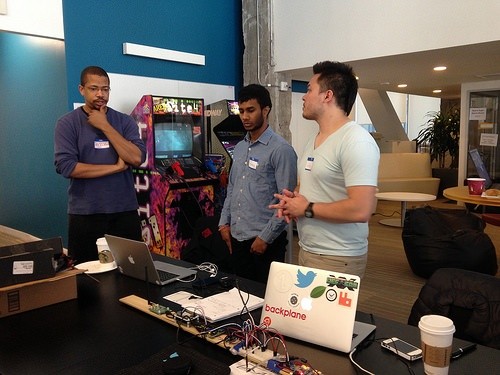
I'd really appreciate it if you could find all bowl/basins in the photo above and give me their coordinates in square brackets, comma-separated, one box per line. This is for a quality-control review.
[466, 178, 487, 196]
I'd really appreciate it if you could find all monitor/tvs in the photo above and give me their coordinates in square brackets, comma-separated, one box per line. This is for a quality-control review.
[153, 121, 194, 159]
[221, 140, 240, 160]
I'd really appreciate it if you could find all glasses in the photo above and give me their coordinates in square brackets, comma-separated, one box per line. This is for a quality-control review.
[84, 85, 112, 93]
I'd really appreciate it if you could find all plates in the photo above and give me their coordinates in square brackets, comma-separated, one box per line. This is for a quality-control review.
[74, 260, 118, 274]
[481, 192, 500, 200]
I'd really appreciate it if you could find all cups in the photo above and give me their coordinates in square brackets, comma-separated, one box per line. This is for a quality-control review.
[96, 237, 116, 268]
[418, 314, 456, 375]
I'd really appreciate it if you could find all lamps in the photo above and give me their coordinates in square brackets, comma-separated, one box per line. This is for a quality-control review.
[123, 42, 206, 66]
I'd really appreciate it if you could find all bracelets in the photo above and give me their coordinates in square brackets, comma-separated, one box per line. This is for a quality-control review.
[219, 225, 228, 231]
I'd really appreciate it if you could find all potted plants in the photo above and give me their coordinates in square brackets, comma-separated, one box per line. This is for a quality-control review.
[417, 106, 460, 198]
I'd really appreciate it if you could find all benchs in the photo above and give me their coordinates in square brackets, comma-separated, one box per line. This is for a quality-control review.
[378, 154, 440, 200]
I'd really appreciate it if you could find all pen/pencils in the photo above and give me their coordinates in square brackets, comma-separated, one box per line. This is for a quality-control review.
[451, 343, 477, 359]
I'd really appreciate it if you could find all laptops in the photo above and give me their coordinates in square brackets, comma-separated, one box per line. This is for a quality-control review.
[469, 149, 500, 190]
[104, 234, 197, 285]
[260, 261, 376, 353]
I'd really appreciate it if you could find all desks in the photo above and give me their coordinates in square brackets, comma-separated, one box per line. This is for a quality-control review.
[371, 192, 436, 227]
[443, 186, 500, 214]
[0, 251, 500, 375]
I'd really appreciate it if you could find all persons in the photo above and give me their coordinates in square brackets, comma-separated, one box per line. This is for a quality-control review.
[268, 60, 381, 279]
[217, 84, 296, 286]
[54, 66, 146, 263]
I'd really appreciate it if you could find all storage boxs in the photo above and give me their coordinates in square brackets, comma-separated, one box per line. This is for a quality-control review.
[0, 225, 88, 319]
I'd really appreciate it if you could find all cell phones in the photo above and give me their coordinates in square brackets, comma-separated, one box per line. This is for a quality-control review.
[381, 337, 423, 361]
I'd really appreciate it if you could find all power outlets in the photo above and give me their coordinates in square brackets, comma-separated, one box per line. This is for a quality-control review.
[279, 80, 290, 92]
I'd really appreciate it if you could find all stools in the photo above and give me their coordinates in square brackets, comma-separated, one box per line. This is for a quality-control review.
[437, 204, 467, 214]
[482, 214, 500, 227]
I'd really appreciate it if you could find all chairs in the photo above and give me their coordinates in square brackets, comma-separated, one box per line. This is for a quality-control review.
[401, 207, 497, 276]
[407, 268, 500, 350]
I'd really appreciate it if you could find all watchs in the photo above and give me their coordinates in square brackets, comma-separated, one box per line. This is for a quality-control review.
[305, 203, 314, 218]
